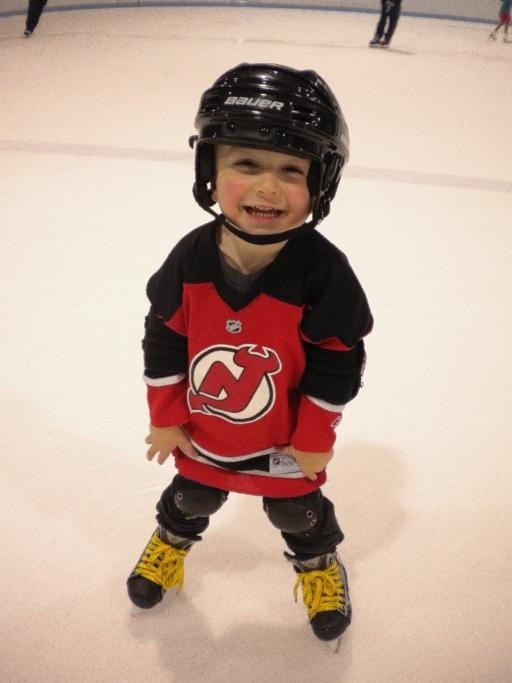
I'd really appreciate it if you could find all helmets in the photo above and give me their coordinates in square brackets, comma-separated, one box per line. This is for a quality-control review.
[190, 62, 350, 223]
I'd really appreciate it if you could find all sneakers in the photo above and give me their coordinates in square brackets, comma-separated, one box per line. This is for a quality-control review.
[128, 516, 202, 609]
[283, 550, 352, 641]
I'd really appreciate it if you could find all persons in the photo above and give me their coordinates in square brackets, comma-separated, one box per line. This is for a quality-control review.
[369, 0, 402, 43]
[492, 1, 512, 40]
[127, 63, 375, 643]
[23, 1, 48, 32]
